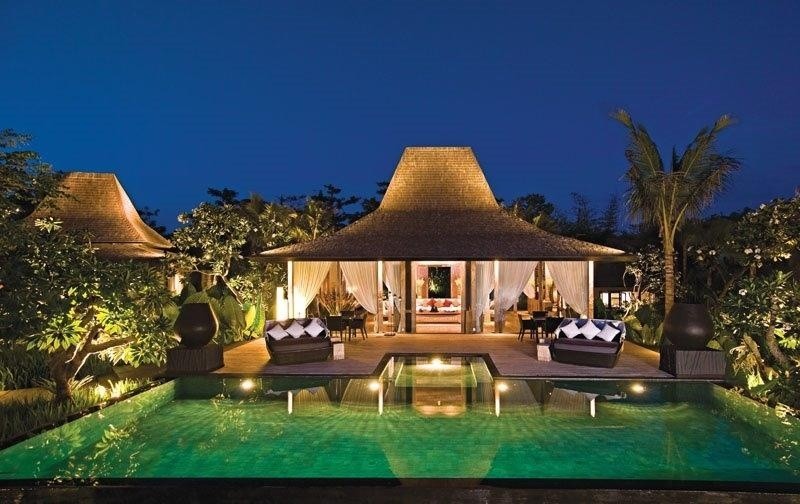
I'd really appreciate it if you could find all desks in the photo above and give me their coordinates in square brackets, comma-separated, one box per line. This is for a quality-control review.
[536, 339, 552, 363]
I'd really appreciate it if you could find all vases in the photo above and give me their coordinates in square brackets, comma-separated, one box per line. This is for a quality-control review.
[173, 302, 220, 346]
[660, 300, 715, 349]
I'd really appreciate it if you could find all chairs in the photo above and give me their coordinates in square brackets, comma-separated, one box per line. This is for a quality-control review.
[325, 310, 369, 342]
[516, 308, 559, 341]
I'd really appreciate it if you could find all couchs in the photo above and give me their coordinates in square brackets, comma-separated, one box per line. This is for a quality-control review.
[550, 316, 626, 369]
[263, 315, 332, 366]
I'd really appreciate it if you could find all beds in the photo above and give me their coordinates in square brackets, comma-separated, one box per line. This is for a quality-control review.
[416, 298, 461, 336]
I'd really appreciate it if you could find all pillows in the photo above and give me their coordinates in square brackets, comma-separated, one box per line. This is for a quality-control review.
[303, 320, 324, 339]
[597, 324, 621, 343]
[579, 318, 601, 340]
[444, 299, 452, 306]
[426, 297, 436, 306]
[561, 322, 580, 338]
[286, 320, 306, 339]
[268, 322, 288, 340]
